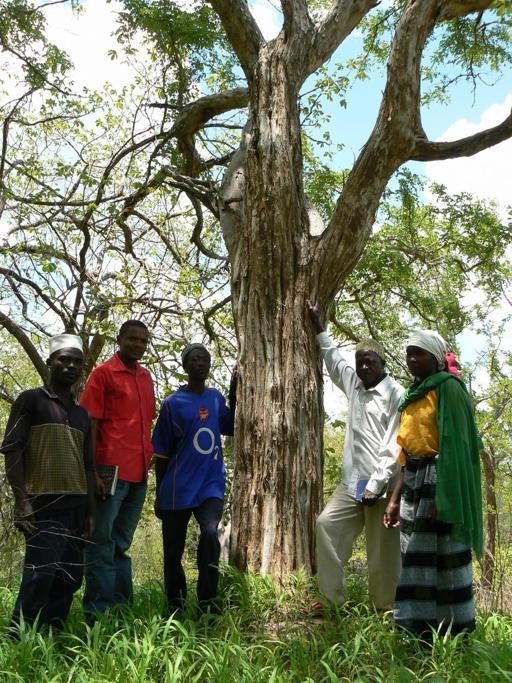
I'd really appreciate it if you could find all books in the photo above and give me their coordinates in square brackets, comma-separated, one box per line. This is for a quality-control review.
[355, 478, 385, 503]
[96, 462, 119, 496]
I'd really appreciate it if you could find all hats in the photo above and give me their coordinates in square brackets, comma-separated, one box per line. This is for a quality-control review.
[181, 343, 207, 363]
[355, 338, 386, 361]
[48, 334, 84, 358]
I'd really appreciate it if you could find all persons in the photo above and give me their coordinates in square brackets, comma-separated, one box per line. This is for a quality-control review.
[378, 327, 487, 652]
[304, 294, 404, 616]
[150, 339, 239, 624]
[78, 316, 157, 632]
[0, 333, 95, 645]
